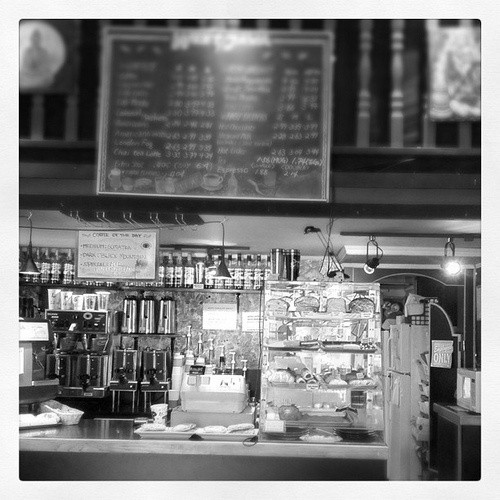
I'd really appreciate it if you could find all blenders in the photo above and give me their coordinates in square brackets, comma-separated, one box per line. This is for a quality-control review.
[44, 310, 171, 399]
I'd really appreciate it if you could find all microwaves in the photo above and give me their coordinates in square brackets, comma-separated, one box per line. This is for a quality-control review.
[456, 366, 480, 413]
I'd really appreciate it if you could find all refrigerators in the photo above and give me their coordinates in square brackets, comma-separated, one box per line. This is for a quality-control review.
[386, 324, 430, 480]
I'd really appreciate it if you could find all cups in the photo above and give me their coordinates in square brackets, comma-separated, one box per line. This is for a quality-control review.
[150, 404, 168, 426]
[271, 248, 285, 279]
[284, 248, 301, 280]
[84, 294, 97, 310]
[71, 295, 83, 310]
[96, 292, 110, 310]
[47, 288, 62, 309]
[60, 292, 73, 310]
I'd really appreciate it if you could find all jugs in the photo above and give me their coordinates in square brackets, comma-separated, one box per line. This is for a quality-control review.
[120, 295, 138, 333]
[137, 296, 157, 333]
[157, 295, 177, 334]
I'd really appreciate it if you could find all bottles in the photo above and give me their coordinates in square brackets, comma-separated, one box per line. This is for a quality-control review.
[19, 247, 74, 284]
[155, 251, 271, 290]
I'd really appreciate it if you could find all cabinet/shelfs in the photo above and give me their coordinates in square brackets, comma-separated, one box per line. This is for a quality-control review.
[260, 278, 382, 432]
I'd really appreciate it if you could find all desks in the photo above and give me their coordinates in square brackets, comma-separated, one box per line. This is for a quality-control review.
[432, 401, 481, 481]
[19, 419, 389, 481]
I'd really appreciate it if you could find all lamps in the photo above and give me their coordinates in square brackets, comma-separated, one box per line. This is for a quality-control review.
[363, 239, 385, 274]
[19, 213, 44, 275]
[439, 239, 463, 279]
[213, 221, 234, 278]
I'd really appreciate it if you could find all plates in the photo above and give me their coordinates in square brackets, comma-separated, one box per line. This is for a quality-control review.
[265, 426, 377, 443]
[196, 428, 260, 442]
[134, 426, 196, 439]
